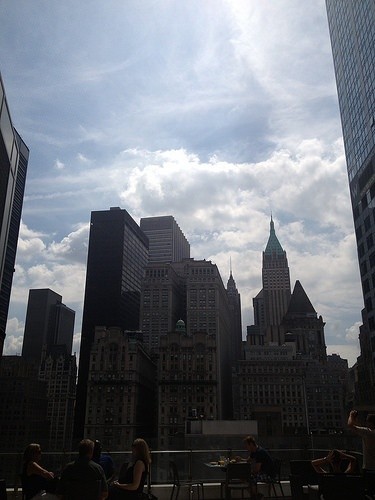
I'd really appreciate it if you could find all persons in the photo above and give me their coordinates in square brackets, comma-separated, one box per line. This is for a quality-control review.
[93, 438, 152, 500]
[234, 435, 276, 500]
[21, 444, 55, 500]
[311, 449, 358, 476]
[347, 410, 375, 500]
[43, 438, 109, 500]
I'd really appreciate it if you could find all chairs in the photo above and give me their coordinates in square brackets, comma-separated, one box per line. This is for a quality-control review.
[256, 457, 286, 500]
[169, 459, 204, 500]
[225, 464, 254, 500]
[109, 462, 152, 500]
[64, 479, 102, 500]
[289, 450, 375, 500]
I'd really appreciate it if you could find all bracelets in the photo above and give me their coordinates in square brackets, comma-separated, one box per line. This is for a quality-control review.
[322, 457, 326, 464]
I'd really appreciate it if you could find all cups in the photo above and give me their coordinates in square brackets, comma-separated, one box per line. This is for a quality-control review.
[220, 456, 225, 467]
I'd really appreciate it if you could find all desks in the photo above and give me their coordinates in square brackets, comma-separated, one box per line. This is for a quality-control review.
[203, 461, 251, 500]
